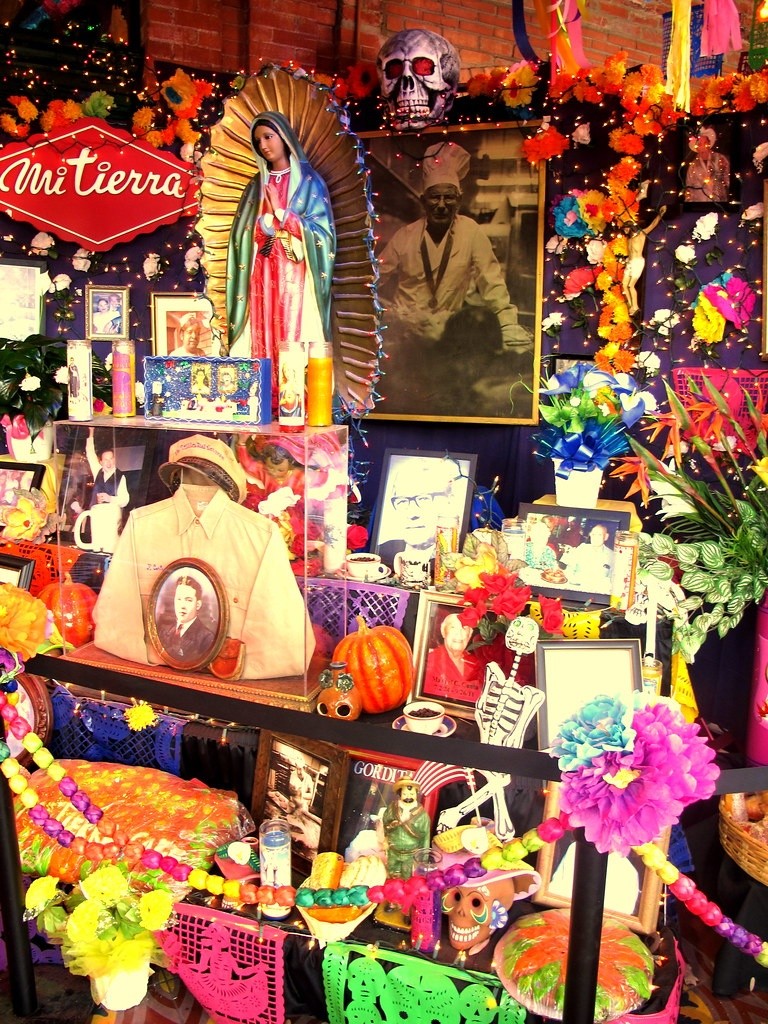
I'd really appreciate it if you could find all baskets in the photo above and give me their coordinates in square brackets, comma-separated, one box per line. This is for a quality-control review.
[719, 793, 768, 887]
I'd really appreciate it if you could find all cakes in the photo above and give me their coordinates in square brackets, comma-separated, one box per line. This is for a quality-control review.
[10, 757, 242, 905]
[501, 911, 655, 1020]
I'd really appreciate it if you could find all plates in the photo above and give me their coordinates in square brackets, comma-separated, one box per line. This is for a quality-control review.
[336, 564, 391, 582]
[392, 714, 457, 737]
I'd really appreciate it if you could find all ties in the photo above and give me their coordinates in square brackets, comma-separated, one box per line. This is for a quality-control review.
[176, 625, 182, 637]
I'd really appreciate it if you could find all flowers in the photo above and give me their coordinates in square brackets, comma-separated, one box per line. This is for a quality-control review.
[547, 683, 720, 860]
[443, 532, 565, 641]
[624, 368, 768, 664]
[24, 859, 178, 1005]
[0, 47, 768, 461]
[530, 363, 661, 478]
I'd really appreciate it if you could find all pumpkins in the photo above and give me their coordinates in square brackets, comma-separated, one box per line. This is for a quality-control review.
[35, 572, 99, 647]
[330, 617, 413, 715]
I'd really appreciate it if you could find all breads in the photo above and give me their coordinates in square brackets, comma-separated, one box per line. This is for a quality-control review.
[310, 851, 388, 923]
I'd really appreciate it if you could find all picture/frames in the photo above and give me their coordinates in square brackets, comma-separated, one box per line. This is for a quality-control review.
[52, 425, 159, 544]
[534, 638, 642, 754]
[150, 293, 222, 357]
[354, 119, 546, 425]
[372, 447, 479, 588]
[0, 257, 47, 342]
[4, 670, 54, 766]
[84, 285, 130, 341]
[516, 502, 630, 606]
[248, 728, 351, 877]
[0, 552, 35, 592]
[404, 589, 538, 721]
[146, 556, 231, 673]
[0, 461, 47, 531]
[336, 743, 440, 860]
[531, 778, 672, 935]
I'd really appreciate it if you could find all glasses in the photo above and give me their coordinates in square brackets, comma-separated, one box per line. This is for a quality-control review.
[391, 492, 446, 510]
[425, 193, 458, 206]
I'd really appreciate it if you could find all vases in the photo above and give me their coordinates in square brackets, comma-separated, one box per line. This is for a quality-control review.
[548, 455, 604, 509]
[94, 955, 151, 1011]
[744, 587, 768, 767]
[9, 419, 55, 463]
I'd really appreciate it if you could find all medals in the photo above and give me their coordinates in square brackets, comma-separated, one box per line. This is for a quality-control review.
[428, 296, 438, 308]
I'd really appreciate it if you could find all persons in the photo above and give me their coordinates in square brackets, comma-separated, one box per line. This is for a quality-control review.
[615, 205, 666, 317]
[443, 304, 534, 421]
[439, 867, 516, 951]
[69, 357, 81, 400]
[373, 171, 533, 416]
[376, 458, 458, 590]
[92, 295, 122, 334]
[0, 469, 37, 506]
[287, 761, 327, 805]
[382, 775, 430, 925]
[161, 575, 216, 662]
[515, 512, 616, 592]
[684, 125, 730, 203]
[84, 426, 129, 543]
[166, 312, 207, 357]
[237, 432, 350, 578]
[225, 110, 338, 420]
[420, 608, 490, 707]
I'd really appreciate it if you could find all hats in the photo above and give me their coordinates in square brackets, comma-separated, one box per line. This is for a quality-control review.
[422, 141, 471, 196]
[393, 776, 421, 792]
[158, 434, 247, 505]
[688, 126, 716, 153]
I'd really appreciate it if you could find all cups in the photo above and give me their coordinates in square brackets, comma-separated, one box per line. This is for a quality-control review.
[338, 553, 381, 578]
[394, 549, 429, 586]
[403, 702, 445, 735]
[74, 503, 122, 553]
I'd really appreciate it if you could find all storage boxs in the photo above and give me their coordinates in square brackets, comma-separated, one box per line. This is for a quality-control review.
[143, 355, 272, 425]
